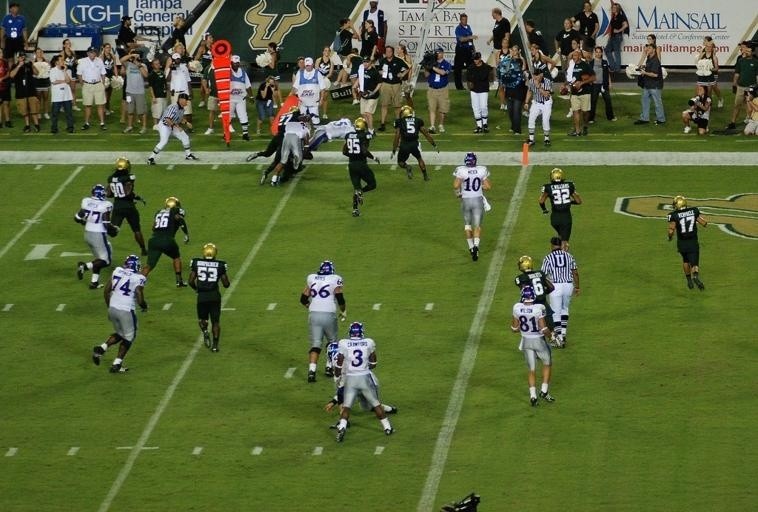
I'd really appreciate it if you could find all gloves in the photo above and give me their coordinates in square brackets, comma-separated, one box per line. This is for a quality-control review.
[140, 305, 149, 313]
[374, 157, 381, 165]
[140, 197, 147, 206]
[390, 149, 396, 159]
[184, 234, 190, 244]
[338, 310, 347, 322]
[435, 147, 441, 153]
[248, 97, 255, 105]
[542, 210, 549, 215]
[456, 190, 462, 198]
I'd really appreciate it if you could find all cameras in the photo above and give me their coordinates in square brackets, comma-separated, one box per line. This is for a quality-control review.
[269, 83, 275, 88]
[688, 95, 702, 106]
[132, 57, 141, 63]
[23, 61, 32, 67]
[636, 65, 645, 71]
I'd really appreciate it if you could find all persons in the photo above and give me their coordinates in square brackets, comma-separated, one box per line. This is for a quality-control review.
[194, 33, 213, 107]
[118, 14, 149, 62]
[360, 1, 384, 53]
[524, 69, 554, 147]
[299, 261, 346, 383]
[92, 255, 149, 374]
[323, 342, 398, 415]
[525, 20, 556, 82]
[147, 58, 167, 125]
[105, 156, 149, 255]
[454, 13, 477, 91]
[171, 16, 186, 54]
[229, 55, 253, 138]
[395, 45, 416, 109]
[334, 18, 360, 87]
[9, 53, 40, 133]
[190, 243, 231, 353]
[561, 39, 583, 117]
[360, 20, 379, 60]
[580, 38, 593, 63]
[390, 105, 438, 182]
[146, 94, 199, 166]
[453, 154, 493, 262]
[423, 47, 452, 134]
[352, 56, 382, 138]
[725, 41, 758, 136]
[494, 39, 512, 110]
[336, 321, 395, 442]
[246, 106, 311, 187]
[537, 168, 583, 253]
[291, 57, 304, 84]
[141, 196, 190, 289]
[681, 86, 711, 135]
[695, 36, 713, 97]
[0, 47, 13, 128]
[345, 54, 362, 105]
[60, 39, 81, 112]
[375, 46, 410, 131]
[486, 8, 511, 66]
[49, 54, 74, 134]
[696, 43, 724, 108]
[602, 3, 629, 74]
[255, 77, 276, 134]
[342, 117, 382, 217]
[540, 237, 581, 349]
[511, 44, 527, 70]
[569, 2, 599, 39]
[293, 57, 324, 130]
[632, 34, 666, 125]
[164, 41, 205, 100]
[0, 3, 29, 61]
[73, 184, 111, 290]
[99, 43, 117, 115]
[306, 115, 357, 152]
[76, 46, 108, 130]
[118, 48, 148, 135]
[570, 52, 594, 137]
[588, 46, 616, 123]
[256, 40, 284, 106]
[554, 18, 585, 85]
[31, 48, 51, 120]
[316, 47, 333, 120]
[504, 46, 527, 135]
[466, 51, 493, 133]
[202, 58, 220, 135]
[511, 286, 558, 406]
[513, 255, 564, 350]
[667, 195, 707, 292]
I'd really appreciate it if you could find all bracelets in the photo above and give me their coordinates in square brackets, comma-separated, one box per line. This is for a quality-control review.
[137, 65, 143, 69]
[525, 103, 529, 104]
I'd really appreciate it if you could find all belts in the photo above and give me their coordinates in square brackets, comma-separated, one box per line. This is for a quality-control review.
[82, 80, 103, 85]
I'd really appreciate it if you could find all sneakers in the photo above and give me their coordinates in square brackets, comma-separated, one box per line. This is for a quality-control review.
[67, 127, 74, 133]
[500, 104, 505, 109]
[33, 124, 40, 132]
[247, 153, 257, 161]
[694, 276, 704, 289]
[271, 180, 277, 186]
[525, 139, 535, 147]
[203, 333, 210, 348]
[726, 123, 737, 130]
[470, 247, 475, 255]
[568, 131, 582, 136]
[472, 247, 479, 262]
[123, 126, 133, 133]
[428, 126, 437, 134]
[385, 427, 393, 435]
[684, 126, 692, 134]
[378, 126, 386, 132]
[204, 128, 215, 136]
[336, 430, 345, 442]
[148, 158, 156, 165]
[718, 99, 724, 108]
[531, 398, 538, 406]
[550, 335, 569, 349]
[325, 366, 334, 377]
[260, 169, 267, 184]
[653, 120, 664, 124]
[186, 154, 199, 161]
[43, 113, 50, 120]
[100, 124, 107, 130]
[438, 124, 445, 133]
[24, 125, 31, 133]
[230, 123, 236, 133]
[177, 280, 187, 288]
[78, 261, 84, 280]
[110, 365, 129, 373]
[242, 133, 252, 141]
[504, 103, 508, 110]
[80, 124, 89, 130]
[634, 120, 649, 125]
[353, 209, 361, 216]
[308, 371, 316, 383]
[139, 127, 148, 133]
[120, 119, 126, 124]
[198, 100, 206, 107]
[688, 281, 695, 289]
[95, 346, 104, 366]
[354, 190, 364, 205]
[211, 343, 218, 352]
[483, 126, 490, 133]
[37, 113, 41, 120]
[142, 249, 148, 256]
[424, 175, 430, 182]
[5, 121, 14, 128]
[544, 135, 551, 146]
[352, 98, 359, 106]
[407, 165, 413, 179]
[90, 282, 105, 289]
[51, 127, 58, 133]
[473, 127, 483, 133]
[540, 391, 555, 401]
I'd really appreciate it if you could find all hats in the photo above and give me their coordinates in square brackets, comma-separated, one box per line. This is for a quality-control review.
[470, 52, 481, 60]
[435, 47, 444, 52]
[9, 2, 21, 7]
[550, 237, 561, 246]
[297, 56, 305, 61]
[179, 93, 193, 101]
[532, 69, 543, 76]
[20, 51, 26, 56]
[120, 16, 133, 21]
[88, 46, 97, 53]
[362, 55, 370, 62]
[305, 57, 314, 66]
[231, 55, 241, 63]
[738, 41, 747, 45]
[172, 53, 182, 60]
[646, 44, 656, 49]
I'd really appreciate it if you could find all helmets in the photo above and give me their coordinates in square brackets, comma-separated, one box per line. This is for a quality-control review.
[288, 105, 299, 114]
[91, 184, 105, 198]
[203, 242, 219, 260]
[464, 152, 476, 166]
[399, 105, 415, 117]
[320, 259, 334, 274]
[114, 156, 130, 172]
[350, 322, 364, 335]
[517, 256, 535, 274]
[327, 342, 338, 359]
[550, 167, 565, 183]
[124, 254, 141, 272]
[522, 285, 537, 302]
[163, 196, 181, 209]
[672, 196, 687, 210]
[353, 117, 367, 131]
